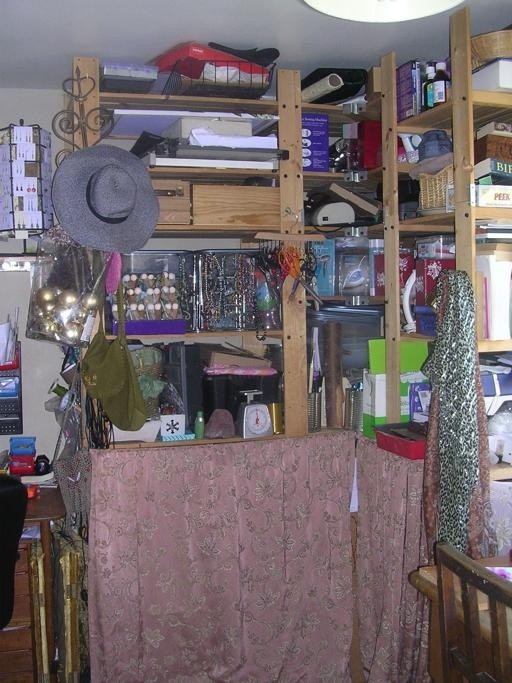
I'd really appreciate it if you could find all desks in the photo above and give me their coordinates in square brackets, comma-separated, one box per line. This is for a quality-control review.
[0, 467, 69, 683]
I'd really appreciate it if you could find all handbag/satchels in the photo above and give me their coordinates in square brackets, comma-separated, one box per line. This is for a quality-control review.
[81, 333, 146, 430]
[53, 453, 92, 517]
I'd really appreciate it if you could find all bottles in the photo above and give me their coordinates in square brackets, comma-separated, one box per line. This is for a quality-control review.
[432, 62, 450, 106]
[193, 410, 206, 440]
[421, 65, 436, 109]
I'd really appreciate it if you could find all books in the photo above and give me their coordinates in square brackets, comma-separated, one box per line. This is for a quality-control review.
[475, 221, 512, 339]
[475, 157, 512, 185]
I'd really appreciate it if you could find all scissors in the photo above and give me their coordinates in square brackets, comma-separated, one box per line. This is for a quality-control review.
[278, 248, 323, 305]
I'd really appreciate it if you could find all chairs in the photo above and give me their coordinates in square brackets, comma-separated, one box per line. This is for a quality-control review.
[429, 539, 510, 681]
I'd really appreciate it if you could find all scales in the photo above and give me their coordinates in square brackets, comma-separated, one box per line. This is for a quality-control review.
[235, 389, 274, 439]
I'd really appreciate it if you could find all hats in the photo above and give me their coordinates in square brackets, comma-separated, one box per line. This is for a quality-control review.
[52, 146, 159, 253]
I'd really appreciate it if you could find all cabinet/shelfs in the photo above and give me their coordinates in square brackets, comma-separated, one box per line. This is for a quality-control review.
[293, 69, 383, 431]
[380, 6, 512, 425]
[73, 59, 298, 448]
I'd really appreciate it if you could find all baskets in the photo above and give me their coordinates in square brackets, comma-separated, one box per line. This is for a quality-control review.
[417, 165, 454, 209]
[469, 29, 511, 67]
